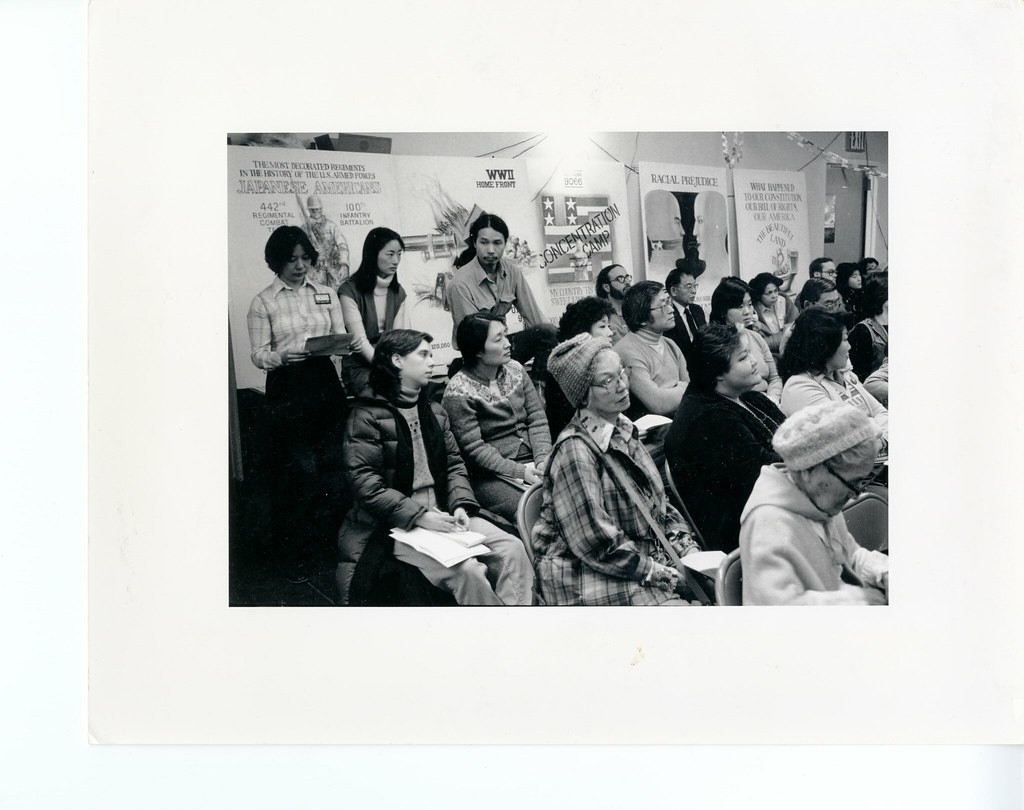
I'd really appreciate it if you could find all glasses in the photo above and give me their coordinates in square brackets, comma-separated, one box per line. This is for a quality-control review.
[813, 301, 842, 313]
[822, 460, 874, 495]
[590, 365, 632, 389]
[675, 284, 698, 290]
[650, 296, 672, 312]
[609, 275, 632, 284]
[821, 269, 837, 276]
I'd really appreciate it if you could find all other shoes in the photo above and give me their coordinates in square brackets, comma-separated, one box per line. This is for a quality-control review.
[287, 557, 308, 583]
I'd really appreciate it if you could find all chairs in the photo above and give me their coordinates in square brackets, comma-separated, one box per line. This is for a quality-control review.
[324, 399, 888, 606]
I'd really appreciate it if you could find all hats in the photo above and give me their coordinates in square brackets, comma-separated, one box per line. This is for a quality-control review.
[771, 400, 874, 472]
[547, 332, 612, 408]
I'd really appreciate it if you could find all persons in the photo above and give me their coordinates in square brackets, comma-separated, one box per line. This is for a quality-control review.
[247, 213, 889, 605]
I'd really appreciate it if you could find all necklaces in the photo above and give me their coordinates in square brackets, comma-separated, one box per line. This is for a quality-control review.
[717, 393, 780, 436]
